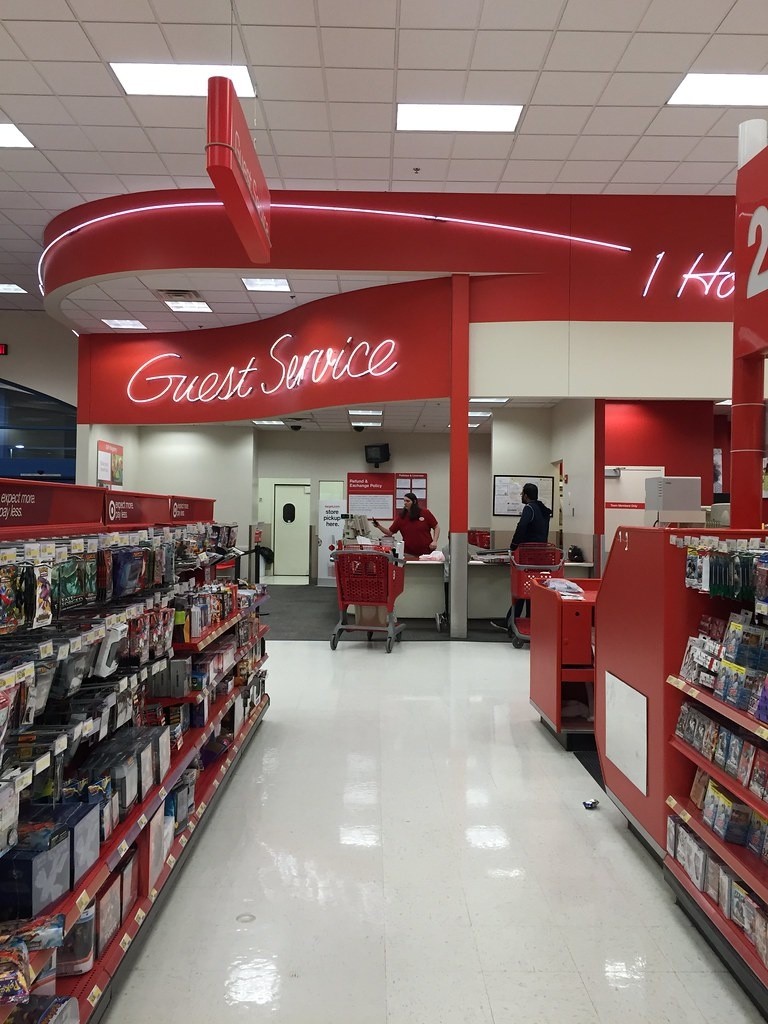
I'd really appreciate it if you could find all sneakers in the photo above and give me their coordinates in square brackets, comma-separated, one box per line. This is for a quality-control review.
[435, 613, 447, 632]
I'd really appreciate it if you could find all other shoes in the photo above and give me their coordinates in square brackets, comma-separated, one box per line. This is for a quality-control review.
[490, 619, 508, 631]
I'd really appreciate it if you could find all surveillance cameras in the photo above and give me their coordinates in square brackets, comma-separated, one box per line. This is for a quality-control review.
[354, 426, 364, 432]
[291, 426, 301, 431]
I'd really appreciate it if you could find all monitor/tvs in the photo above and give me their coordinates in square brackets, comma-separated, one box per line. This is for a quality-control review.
[365, 443, 390, 463]
[351, 514, 369, 536]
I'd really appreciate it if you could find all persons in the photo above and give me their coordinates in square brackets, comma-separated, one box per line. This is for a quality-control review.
[371, 493, 440, 559]
[434, 531, 471, 633]
[491, 483, 550, 631]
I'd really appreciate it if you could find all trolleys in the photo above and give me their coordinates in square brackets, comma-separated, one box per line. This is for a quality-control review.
[508, 540, 566, 651]
[327, 539, 407, 654]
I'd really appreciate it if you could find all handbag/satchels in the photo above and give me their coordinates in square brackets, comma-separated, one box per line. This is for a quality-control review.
[568, 545, 583, 562]
